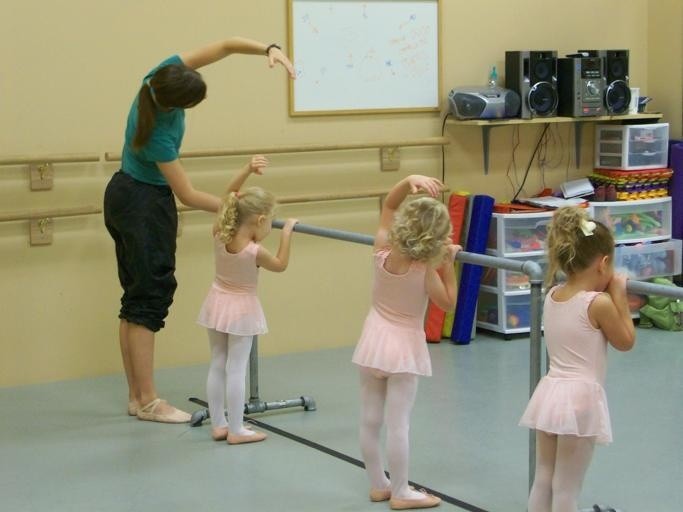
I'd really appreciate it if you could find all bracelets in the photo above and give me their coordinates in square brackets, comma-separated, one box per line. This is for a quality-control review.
[266, 43, 281, 56]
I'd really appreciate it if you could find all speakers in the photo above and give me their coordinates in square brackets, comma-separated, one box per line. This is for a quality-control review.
[505, 51, 559, 119]
[578, 49, 631, 115]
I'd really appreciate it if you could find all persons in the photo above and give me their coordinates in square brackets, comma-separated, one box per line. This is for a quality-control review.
[197, 155, 300, 445]
[516, 204, 637, 512]
[102, 36, 297, 425]
[351, 173, 464, 510]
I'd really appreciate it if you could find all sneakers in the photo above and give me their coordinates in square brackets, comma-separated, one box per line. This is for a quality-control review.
[128, 400, 192, 423]
[392, 493, 442, 508]
[214, 427, 266, 444]
[370, 488, 393, 501]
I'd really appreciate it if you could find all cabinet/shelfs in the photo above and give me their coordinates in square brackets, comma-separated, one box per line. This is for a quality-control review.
[478, 197, 683, 336]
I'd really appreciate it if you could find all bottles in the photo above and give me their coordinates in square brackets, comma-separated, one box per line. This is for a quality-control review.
[490, 66, 496, 80]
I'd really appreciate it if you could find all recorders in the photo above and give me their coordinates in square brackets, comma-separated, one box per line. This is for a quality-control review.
[449, 87, 520, 119]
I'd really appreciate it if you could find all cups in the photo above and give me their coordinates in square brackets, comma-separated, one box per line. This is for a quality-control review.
[629, 87, 640, 115]
[638, 104, 646, 112]
